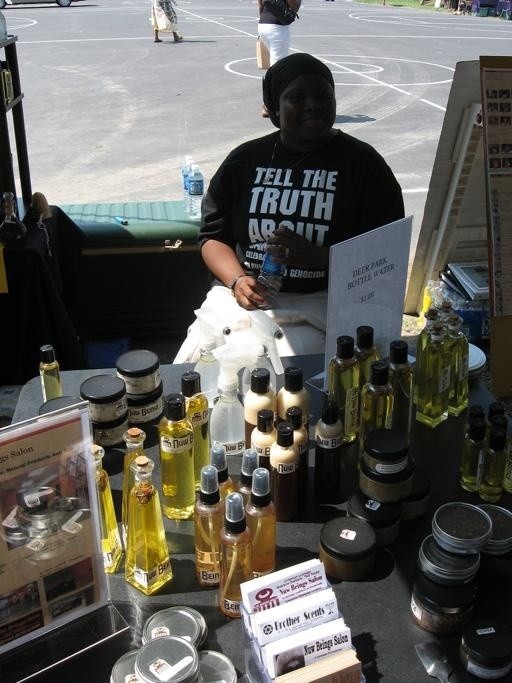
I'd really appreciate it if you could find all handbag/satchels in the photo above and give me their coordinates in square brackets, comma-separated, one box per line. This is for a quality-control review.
[264, 0, 301, 24]
[256, 38, 271, 69]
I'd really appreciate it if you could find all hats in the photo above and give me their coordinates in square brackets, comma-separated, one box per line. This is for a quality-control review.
[260, 50, 336, 127]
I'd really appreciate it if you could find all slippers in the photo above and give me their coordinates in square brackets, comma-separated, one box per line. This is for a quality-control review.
[174, 37, 182, 41]
[154, 40, 162, 42]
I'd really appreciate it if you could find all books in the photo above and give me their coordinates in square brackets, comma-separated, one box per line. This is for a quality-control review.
[436, 260, 489, 302]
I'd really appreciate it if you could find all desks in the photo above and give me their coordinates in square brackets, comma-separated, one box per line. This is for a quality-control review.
[8, 330, 509, 683]
[52, 200, 204, 226]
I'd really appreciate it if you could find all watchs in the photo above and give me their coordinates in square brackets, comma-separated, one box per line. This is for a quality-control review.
[226, 275, 245, 298]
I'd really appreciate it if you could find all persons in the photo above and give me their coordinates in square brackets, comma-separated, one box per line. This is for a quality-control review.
[149, 0, 184, 43]
[171, 54, 405, 368]
[257, 0, 302, 117]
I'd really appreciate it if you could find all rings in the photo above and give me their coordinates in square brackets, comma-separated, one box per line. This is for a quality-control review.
[284, 248, 290, 259]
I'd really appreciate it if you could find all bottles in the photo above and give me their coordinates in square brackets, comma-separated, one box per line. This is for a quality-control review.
[191, 439, 279, 616]
[459, 401, 511, 501]
[195, 344, 243, 442]
[416, 300, 471, 423]
[256, 224, 288, 300]
[86, 428, 176, 598]
[328, 327, 416, 448]
[39, 343, 62, 400]
[181, 155, 205, 220]
[244, 367, 347, 514]
[158, 370, 209, 520]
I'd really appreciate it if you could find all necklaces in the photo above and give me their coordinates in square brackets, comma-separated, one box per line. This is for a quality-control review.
[262, 137, 332, 183]
